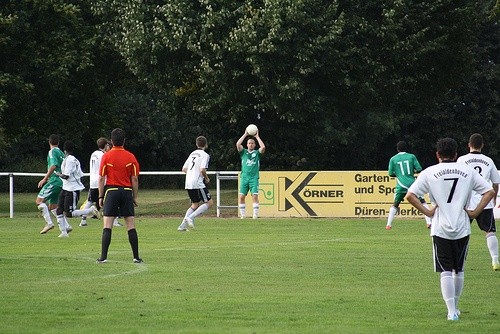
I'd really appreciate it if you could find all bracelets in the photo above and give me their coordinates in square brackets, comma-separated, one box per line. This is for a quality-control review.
[98, 196, 104, 199]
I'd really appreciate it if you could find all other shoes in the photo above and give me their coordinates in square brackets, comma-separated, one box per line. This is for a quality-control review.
[427, 224, 431, 228]
[386, 226, 391, 230]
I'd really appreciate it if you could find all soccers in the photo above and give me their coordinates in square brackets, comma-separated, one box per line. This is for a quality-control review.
[246, 124, 258, 135]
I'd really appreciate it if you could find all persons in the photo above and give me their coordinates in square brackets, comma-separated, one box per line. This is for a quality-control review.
[56, 140, 102, 238]
[78, 138, 123, 227]
[455, 132, 500, 271]
[385, 141, 431, 230]
[36, 134, 73, 234]
[177, 136, 214, 231]
[95, 128, 144, 263]
[236, 128, 265, 219]
[406, 137, 496, 322]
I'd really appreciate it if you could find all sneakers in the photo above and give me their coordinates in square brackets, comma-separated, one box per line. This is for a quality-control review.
[133, 259, 143, 263]
[96, 258, 107, 263]
[185, 218, 194, 229]
[40, 224, 54, 234]
[79, 220, 87, 226]
[66, 225, 72, 233]
[58, 233, 69, 238]
[113, 219, 123, 226]
[447, 310, 460, 321]
[90, 205, 100, 220]
[492, 262, 500, 271]
[177, 228, 189, 231]
